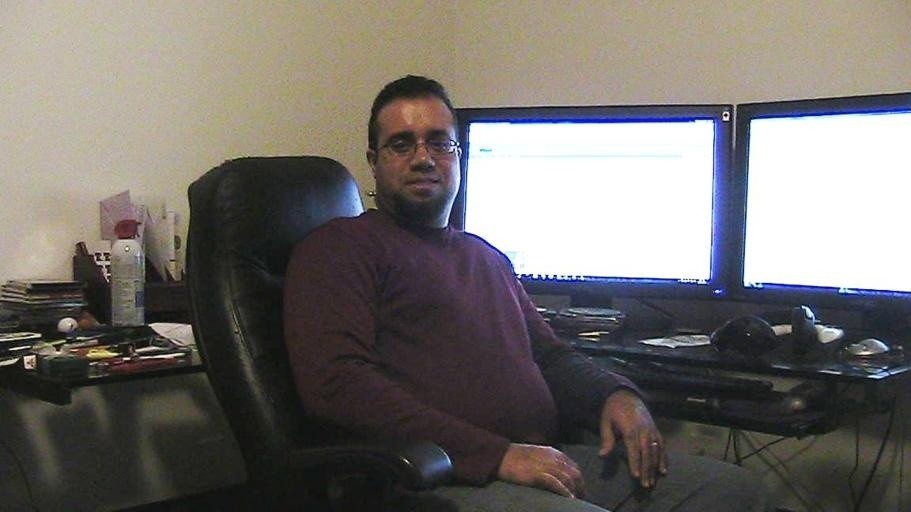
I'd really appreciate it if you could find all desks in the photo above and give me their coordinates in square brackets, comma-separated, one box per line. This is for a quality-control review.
[0, 283, 910, 440]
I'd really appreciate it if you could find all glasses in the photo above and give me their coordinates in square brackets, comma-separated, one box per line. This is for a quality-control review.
[377, 139, 459, 156]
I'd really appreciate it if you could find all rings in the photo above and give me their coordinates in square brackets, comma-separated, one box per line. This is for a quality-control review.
[649, 440, 660, 446]
[560, 458, 568, 468]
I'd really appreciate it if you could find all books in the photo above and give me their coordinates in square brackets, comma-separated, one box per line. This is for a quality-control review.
[0, 278, 90, 309]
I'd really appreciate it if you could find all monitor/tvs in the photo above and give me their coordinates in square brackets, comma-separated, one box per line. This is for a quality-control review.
[451, 103, 729, 333]
[729, 93, 910, 359]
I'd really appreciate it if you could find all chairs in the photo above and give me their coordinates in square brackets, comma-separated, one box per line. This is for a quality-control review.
[183, 155, 452, 509]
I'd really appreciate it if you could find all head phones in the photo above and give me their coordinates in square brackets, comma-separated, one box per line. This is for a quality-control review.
[712, 306, 815, 362]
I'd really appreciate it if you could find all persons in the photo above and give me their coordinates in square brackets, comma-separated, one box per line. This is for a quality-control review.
[278, 73, 770, 511]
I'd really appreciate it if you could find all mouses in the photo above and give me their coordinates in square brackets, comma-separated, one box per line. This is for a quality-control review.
[780, 383, 821, 411]
[846, 338, 890, 354]
